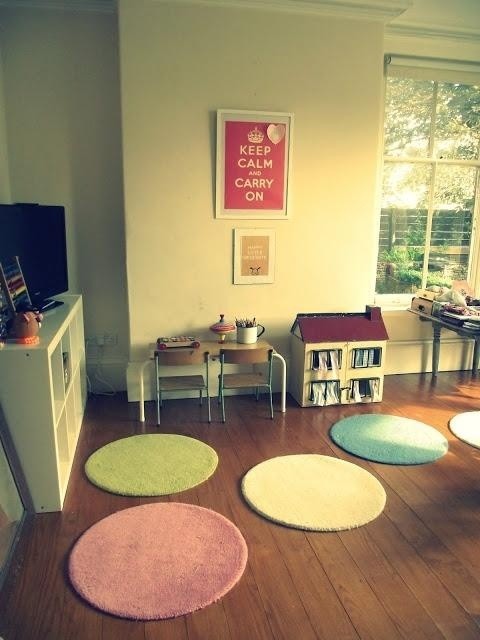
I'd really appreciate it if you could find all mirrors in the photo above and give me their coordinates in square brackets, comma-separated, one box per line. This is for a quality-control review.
[0, 404, 37, 590]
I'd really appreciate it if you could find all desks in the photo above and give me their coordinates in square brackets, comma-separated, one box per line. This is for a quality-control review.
[138, 338, 286, 423]
[406, 308, 480, 377]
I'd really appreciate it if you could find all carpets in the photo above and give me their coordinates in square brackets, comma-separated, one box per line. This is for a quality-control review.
[330, 414, 449, 466]
[240, 453, 387, 533]
[449, 409, 479, 450]
[84, 433, 219, 497]
[66, 502, 249, 622]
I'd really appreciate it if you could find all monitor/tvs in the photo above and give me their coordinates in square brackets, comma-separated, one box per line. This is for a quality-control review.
[0, 204, 69, 312]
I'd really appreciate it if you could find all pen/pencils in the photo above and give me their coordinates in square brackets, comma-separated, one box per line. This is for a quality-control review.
[235, 316, 257, 328]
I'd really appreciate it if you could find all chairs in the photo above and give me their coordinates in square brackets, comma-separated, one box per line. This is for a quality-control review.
[218, 348, 274, 422]
[154, 350, 211, 425]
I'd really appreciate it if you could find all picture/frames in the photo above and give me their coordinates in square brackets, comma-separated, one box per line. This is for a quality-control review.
[215, 108, 295, 221]
[232, 227, 275, 285]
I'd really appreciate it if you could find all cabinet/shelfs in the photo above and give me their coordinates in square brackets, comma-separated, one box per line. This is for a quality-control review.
[0, 294, 88, 515]
